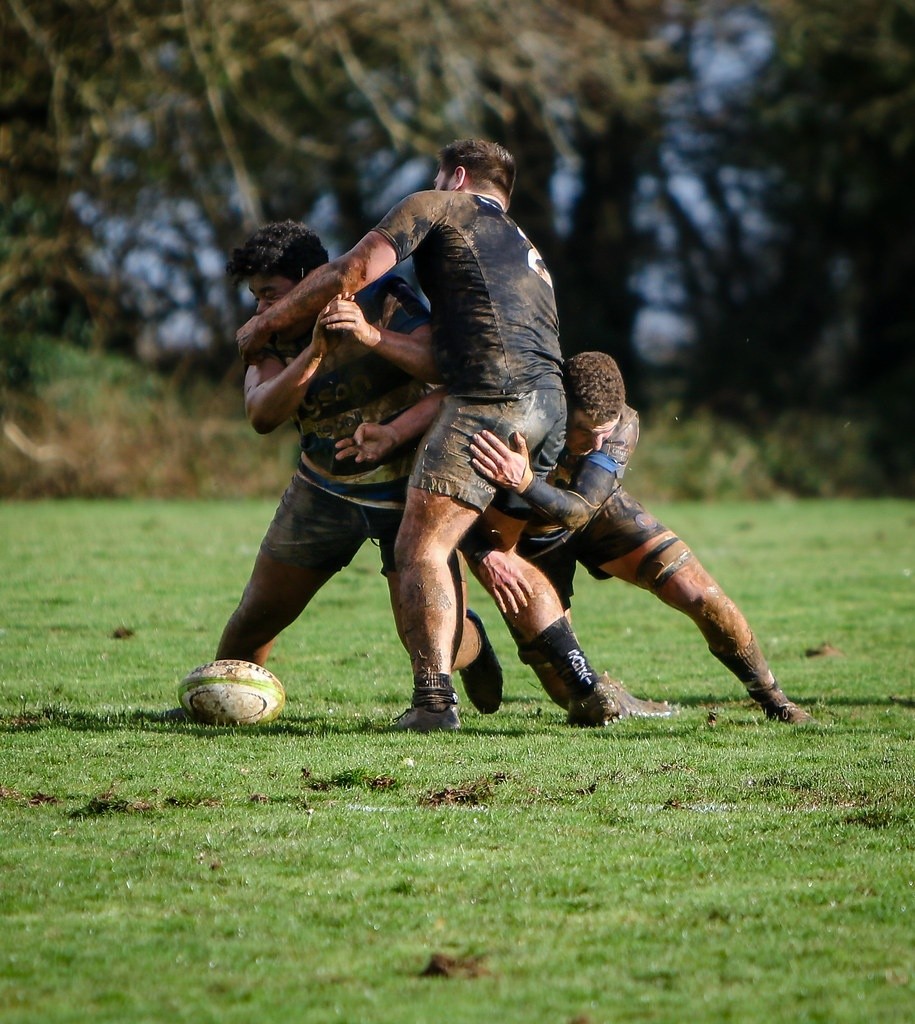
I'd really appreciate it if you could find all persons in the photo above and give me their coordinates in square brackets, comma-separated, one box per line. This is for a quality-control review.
[232, 135, 675, 736]
[457, 349, 816, 724]
[211, 219, 504, 714]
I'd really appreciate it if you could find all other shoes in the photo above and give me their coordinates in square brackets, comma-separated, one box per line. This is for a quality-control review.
[383, 708, 461, 731]
[765, 702, 817, 724]
[459, 608, 503, 715]
[566, 692, 673, 721]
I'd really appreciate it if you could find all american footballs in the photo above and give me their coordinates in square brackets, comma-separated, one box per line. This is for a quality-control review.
[178, 659, 286, 724]
